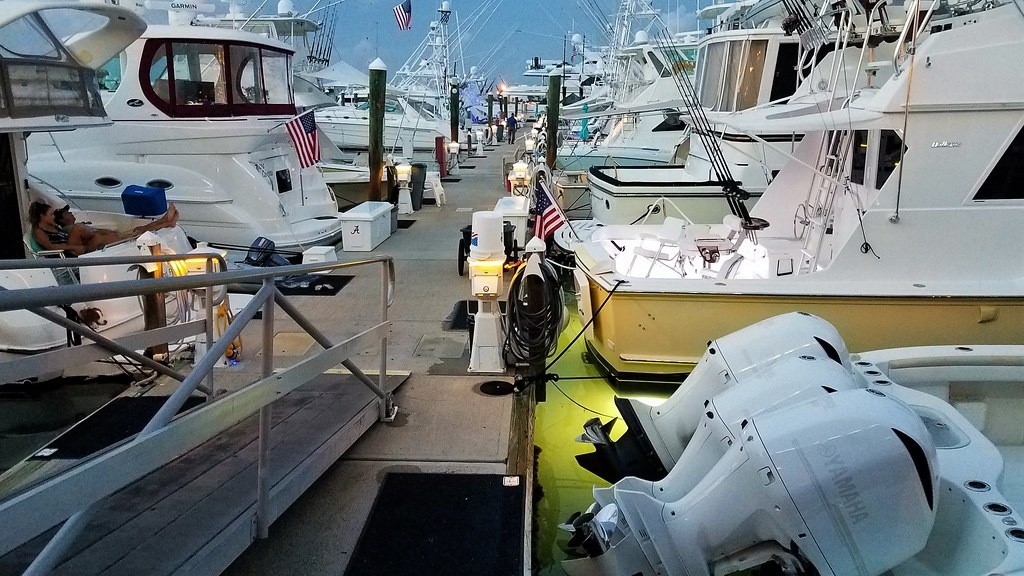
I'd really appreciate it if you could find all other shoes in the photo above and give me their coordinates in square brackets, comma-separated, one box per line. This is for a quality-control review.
[511, 143, 514, 144]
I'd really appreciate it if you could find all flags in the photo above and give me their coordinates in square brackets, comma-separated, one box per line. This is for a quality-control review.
[570, 46, 577, 62]
[286, 109, 322, 169]
[393, 0, 412, 30]
[533, 182, 565, 241]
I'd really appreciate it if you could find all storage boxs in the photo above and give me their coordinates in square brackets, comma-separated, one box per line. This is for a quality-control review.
[494, 194, 529, 246]
[340, 201, 395, 253]
[121, 184, 168, 216]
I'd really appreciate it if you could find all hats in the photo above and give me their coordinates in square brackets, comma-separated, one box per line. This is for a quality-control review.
[511, 113, 514, 115]
[53, 205, 70, 224]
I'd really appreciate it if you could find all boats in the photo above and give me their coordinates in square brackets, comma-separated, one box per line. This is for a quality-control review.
[23, 25, 346, 272]
[495, 2, 1024, 390]
[0, 0, 256, 390]
[140, 0, 514, 214]
[552, 313, 1024, 575]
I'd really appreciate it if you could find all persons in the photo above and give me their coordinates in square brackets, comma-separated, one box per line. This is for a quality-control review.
[506, 113, 517, 144]
[28, 202, 179, 258]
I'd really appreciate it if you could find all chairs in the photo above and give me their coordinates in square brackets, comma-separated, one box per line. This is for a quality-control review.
[583, 132, 601, 151]
[23, 231, 80, 284]
[689, 214, 746, 270]
[701, 252, 744, 280]
[625, 217, 692, 279]
